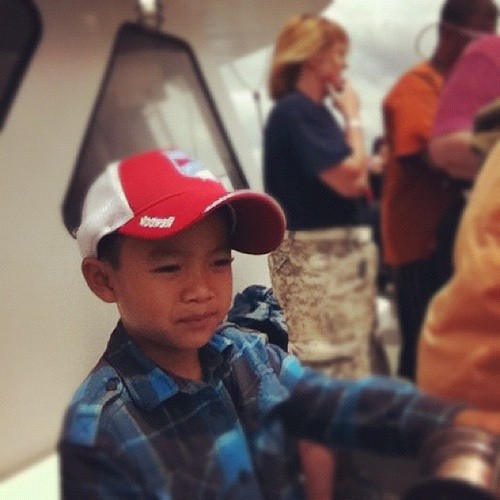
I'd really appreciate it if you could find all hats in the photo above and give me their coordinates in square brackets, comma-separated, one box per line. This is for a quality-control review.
[76, 150, 287, 262]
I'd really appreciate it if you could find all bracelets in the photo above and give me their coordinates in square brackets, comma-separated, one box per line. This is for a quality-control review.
[343, 118, 362, 129]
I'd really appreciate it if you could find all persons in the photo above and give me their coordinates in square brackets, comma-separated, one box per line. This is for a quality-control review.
[370, 0, 500, 383]
[415, 141, 500, 413]
[262, 15, 378, 500]
[59, 152, 500, 500]
